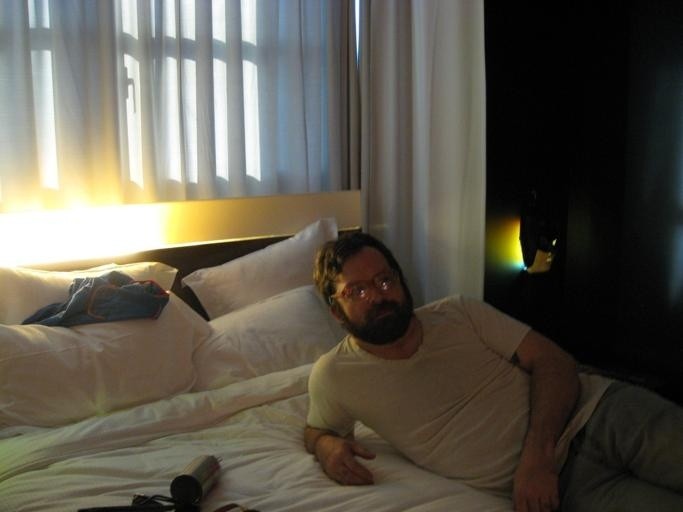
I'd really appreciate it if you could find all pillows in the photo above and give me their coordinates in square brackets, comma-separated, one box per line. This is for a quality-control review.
[193, 282, 347, 396]
[1, 259, 182, 326]
[181, 217, 342, 324]
[1, 289, 212, 433]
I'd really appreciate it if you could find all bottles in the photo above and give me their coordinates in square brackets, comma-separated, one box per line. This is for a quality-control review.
[170, 453, 224, 506]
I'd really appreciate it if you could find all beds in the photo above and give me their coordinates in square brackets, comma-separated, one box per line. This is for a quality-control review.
[0, 189, 514, 512]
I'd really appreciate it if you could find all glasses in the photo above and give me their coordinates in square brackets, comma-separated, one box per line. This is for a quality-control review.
[329, 274, 406, 304]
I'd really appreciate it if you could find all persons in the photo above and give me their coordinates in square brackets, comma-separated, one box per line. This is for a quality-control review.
[303, 226, 683, 511]
